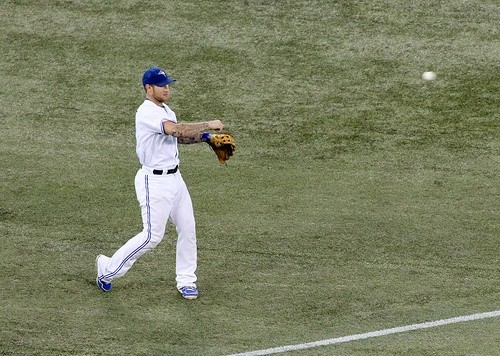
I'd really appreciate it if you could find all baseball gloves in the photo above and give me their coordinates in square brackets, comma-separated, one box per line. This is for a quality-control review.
[208, 133, 236, 167]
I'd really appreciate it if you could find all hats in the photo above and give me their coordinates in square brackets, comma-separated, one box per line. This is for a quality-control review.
[142, 67, 177, 87]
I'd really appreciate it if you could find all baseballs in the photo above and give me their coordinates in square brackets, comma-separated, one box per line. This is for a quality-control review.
[422, 71, 436, 81]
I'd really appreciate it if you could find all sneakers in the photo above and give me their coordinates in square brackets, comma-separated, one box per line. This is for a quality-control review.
[178, 286, 198, 299]
[95, 255, 112, 292]
